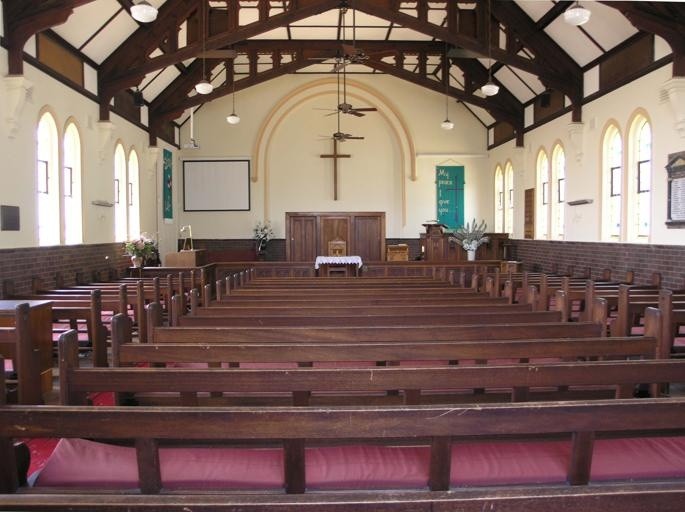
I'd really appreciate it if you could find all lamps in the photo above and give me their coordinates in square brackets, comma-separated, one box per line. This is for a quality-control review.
[439, 42, 455, 132]
[481, 0, 500, 96]
[194, 0, 214, 94]
[564, 0, 591, 25]
[129, 0, 158, 23]
[227, 70, 240, 125]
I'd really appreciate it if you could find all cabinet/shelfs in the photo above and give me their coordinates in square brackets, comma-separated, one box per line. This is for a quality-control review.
[387, 245, 408, 261]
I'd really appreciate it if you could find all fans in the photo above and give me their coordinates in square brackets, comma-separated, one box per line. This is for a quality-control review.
[307, 0, 371, 74]
[315, 3, 377, 117]
[317, 55, 365, 143]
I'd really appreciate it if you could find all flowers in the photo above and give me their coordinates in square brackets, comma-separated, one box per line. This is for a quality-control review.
[122, 234, 153, 256]
[448, 218, 489, 251]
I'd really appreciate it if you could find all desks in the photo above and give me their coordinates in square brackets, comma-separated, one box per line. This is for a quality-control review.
[318, 256, 358, 277]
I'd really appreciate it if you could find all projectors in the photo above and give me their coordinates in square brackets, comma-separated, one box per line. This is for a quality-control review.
[182, 139, 200, 149]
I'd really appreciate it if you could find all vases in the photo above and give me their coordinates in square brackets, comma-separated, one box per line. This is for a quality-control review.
[467, 248, 475, 261]
[131, 255, 143, 268]
